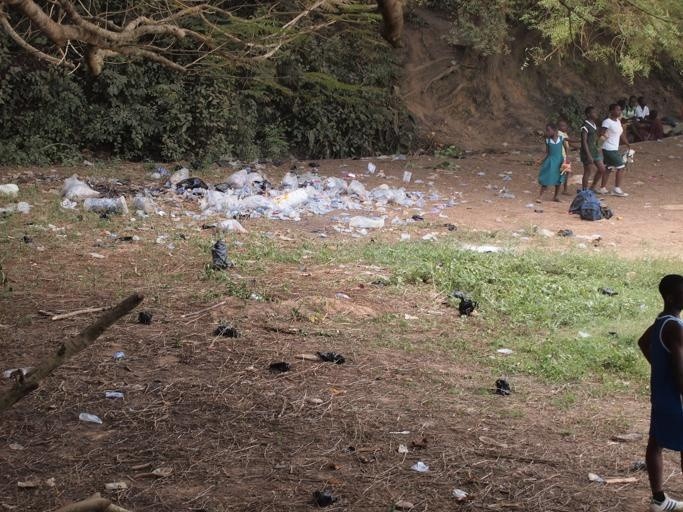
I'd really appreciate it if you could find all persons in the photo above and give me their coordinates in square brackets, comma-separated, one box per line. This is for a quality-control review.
[538, 104, 639, 202]
[616, 95, 683, 143]
[638, 274, 683, 512]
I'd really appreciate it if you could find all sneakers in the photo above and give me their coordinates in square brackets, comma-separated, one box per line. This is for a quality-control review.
[649, 492, 683, 512]
[611, 187, 629, 197]
[597, 187, 609, 194]
